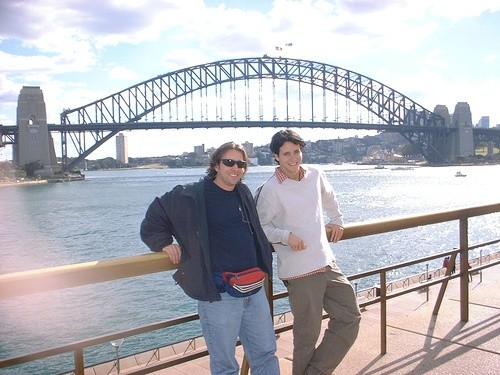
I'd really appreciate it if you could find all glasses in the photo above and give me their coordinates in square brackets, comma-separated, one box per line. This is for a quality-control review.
[219, 159, 246, 168]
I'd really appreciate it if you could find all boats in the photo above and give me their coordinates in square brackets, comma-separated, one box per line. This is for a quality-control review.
[391, 167, 413, 170]
[374, 165, 387, 169]
[455, 172, 467, 177]
[47, 171, 86, 183]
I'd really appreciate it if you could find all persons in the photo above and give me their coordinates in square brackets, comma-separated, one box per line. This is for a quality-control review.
[140, 141, 281, 375]
[256, 129, 361, 375]
[443, 256, 455, 273]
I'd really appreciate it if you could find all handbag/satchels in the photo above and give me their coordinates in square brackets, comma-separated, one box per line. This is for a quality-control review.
[222, 267, 266, 297]
